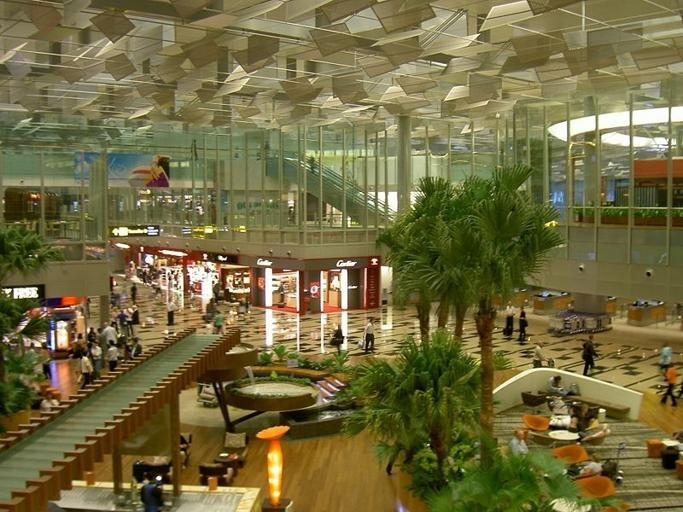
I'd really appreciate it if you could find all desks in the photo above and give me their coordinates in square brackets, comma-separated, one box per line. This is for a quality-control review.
[493, 279, 668, 328]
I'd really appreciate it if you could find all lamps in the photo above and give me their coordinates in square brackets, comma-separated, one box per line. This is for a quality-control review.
[254, 425, 294, 511]
[207, 476, 218, 489]
[84, 471, 95, 485]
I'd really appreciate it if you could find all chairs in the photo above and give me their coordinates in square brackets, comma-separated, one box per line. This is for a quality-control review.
[0, 212, 79, 242]
[515, 387, 631, 511]
[199, 463, 233, 486]
[179, 432, 191, 469]
[219, 431, 251, 468]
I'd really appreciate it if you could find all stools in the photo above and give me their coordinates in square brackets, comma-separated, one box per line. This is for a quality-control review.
[641, 429, 682, 481]
[132, 459, 173, 484]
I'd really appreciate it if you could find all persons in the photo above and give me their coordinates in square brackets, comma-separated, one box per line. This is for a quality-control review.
[71, 301, 141, 389]
[139, 469, 163, 512]
[361, 318, 376, 354]
[202, 278, 235, 336]
[29, 342, 52, 380]
[238, 295, 249, 321]
[187, 272, 190, 286]
[328, 323, 344, 356]
[128, 259, 156, 288]
[110, 288, 116, 310]
[131, 283, 138, 304]
[502, 299, 683, 481]
[166, 296, 176, 326]
[280, 281, 286, 305]
[236, 278, 244, 285]
[167, 271, 179, 289]
[190, 281, 196, 294]
[31, 392, 59, 411]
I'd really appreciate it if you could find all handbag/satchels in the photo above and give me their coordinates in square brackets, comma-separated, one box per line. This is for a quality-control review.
[358, 339, 365, 350]
[330, 338, 336, 346]
